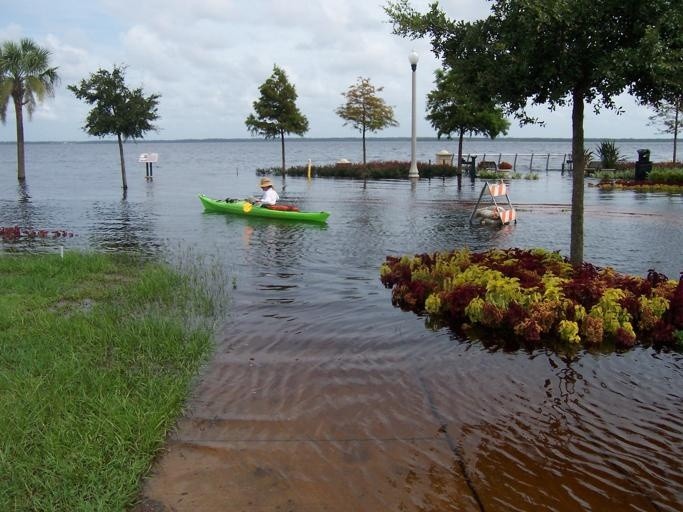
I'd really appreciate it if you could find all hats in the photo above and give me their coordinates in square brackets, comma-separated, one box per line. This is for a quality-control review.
[260, 178, 272, 187]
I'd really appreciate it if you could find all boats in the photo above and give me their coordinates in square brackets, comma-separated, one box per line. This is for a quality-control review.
[196, 191, 333, 224]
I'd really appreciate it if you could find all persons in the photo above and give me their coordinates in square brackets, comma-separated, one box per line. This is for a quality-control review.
[248, 178, 279, 208]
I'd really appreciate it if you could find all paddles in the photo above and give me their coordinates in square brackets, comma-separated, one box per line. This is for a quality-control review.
[242, 199, 261, 213]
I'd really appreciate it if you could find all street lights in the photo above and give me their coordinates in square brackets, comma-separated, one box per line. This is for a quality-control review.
[404, 44, 423, 180]
